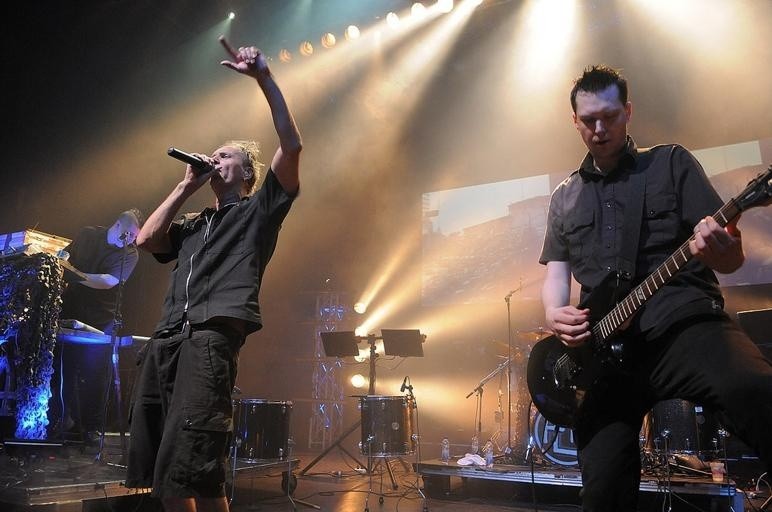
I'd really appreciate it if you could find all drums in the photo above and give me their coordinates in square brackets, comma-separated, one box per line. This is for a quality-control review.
[232, 398, 292, 463]
[533, 411, 581, 467]
[359, 395, 418, 457]
[649, 399, 725, 457]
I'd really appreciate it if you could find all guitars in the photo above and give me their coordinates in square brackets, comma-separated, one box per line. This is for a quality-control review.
[526, 168, 772, 428]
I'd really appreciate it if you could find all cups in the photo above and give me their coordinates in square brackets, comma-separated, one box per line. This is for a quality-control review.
[710, 462, 725, 482]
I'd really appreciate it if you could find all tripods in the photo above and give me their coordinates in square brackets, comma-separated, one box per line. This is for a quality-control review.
[492, 297, 546, 468]
[299, 353, 409, 490]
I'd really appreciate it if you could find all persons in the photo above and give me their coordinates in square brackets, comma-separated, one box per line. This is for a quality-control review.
[538, 65, 771, 512]
[50, 202, 141, 457]
[123, 32, 301, 511]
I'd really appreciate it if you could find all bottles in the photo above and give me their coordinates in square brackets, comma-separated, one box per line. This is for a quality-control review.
[484, 439, 494, 470]
[471, 433, 479, 454]
[441, 436, 450, 462]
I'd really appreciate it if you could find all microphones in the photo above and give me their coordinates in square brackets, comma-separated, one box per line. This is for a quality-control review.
[118, 229, 130, 241]
[400, 377, 408, 393]
[168, 144, 216, 174]
